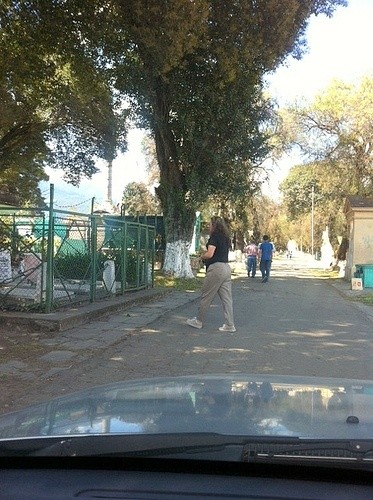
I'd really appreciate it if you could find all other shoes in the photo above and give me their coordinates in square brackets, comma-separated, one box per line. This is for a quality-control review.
[247, 274, 255, 278]
[261, 277, 268, 283]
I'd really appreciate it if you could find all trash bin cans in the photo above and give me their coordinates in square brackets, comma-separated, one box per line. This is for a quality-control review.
[354, 264, 373, 288]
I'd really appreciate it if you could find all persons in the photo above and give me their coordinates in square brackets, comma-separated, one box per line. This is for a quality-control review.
[187, 217, 236, 332]
[259, 235, 276, 283]
[246, 239, 259, 278]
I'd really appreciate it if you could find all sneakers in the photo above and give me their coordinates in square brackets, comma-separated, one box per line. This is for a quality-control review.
[187, 317, 202, 329]
[218, 324, 236, 332]
